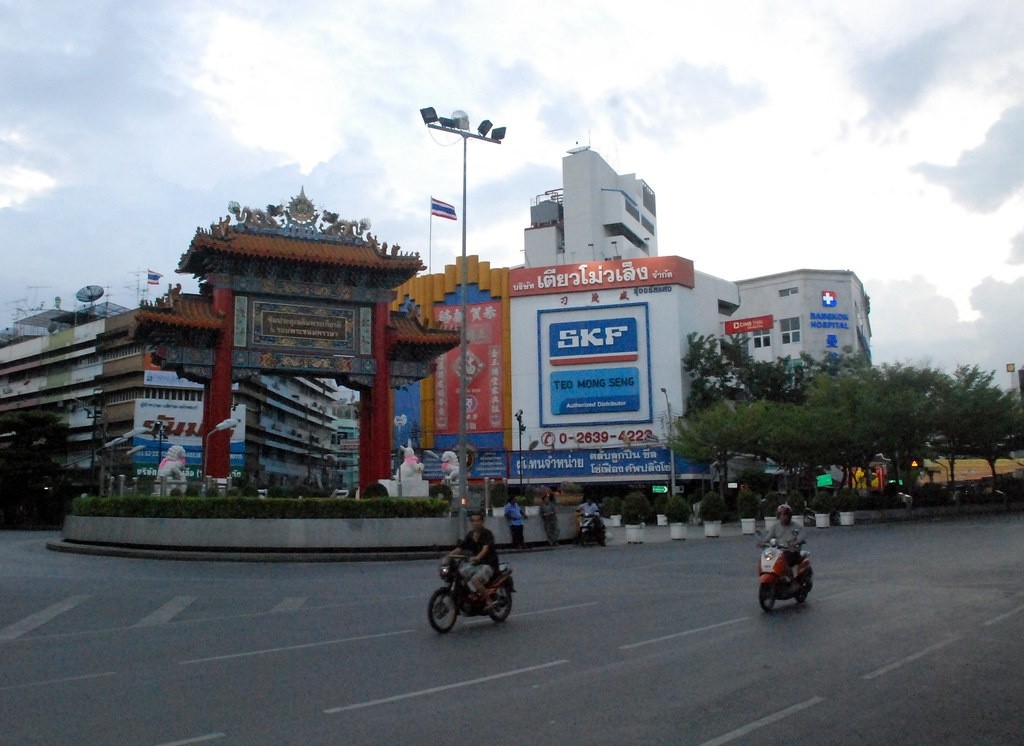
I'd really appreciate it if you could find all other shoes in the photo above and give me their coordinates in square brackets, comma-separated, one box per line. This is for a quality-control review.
[791, 583, 799, 592]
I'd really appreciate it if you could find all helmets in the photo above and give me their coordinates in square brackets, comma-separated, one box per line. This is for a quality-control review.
[776, 504, 792, 521]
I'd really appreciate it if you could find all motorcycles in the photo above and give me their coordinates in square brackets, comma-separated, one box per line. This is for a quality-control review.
[427, 555, 517, 633]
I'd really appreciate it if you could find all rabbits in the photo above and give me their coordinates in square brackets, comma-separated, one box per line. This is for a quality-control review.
[393, 438, 425, 483]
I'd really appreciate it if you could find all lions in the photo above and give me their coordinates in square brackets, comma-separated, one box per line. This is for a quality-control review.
[157, 445, 186, 479]
[441, 451, 459, 484]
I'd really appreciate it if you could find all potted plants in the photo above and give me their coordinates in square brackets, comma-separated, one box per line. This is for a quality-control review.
[788, 490, 806, 527]
[620, 491, 653, 543]
[811, 490, 835, 528]
[762, 491, 785, 530]
[664, 494, 692, 540]
[736, 489, 761, 535]
[599, 497, 623, 527]
[654, 496, 669, 526]
[834, 486, 859, 525]
[698, 491, 725, 537]
[532, 480, 585, 506]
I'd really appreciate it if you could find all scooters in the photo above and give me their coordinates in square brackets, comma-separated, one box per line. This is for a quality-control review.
[754, 529, 813, 612]
[802, 500, 841, 526]
[575, 509, 606, 548]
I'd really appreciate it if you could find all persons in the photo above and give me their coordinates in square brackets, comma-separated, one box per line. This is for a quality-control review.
[446, 513, 499, 610]
[539, 494, 561, 547]
[755, 505, 800, 592]
[504, 494, 528, 549]
[576, 496, 605, 546]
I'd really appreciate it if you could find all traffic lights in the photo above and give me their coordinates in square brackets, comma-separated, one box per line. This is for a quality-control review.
[911, 460, 919, 467]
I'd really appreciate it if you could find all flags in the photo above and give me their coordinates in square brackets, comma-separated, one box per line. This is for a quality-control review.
[432, 198, 457, 220]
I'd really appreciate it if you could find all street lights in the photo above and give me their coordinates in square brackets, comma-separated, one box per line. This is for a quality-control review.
[396, 418, 403, 497]
[89, 437, 129, 496]
[203, 419, 239, 484]
[153, 422, 169, 464]
[514, 409, 526, 496]
[83, 388, 104, 496]
[420, 107, 506, 540]
[108, 427, 148, 498]
[661, 388, 675, 497]
[117, 445, 146, 475]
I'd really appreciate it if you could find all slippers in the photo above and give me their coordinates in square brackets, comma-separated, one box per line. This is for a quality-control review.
[484, 599, 499, 611]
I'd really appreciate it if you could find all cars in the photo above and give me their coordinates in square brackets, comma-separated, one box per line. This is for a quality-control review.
[965, 485, 1006, 502]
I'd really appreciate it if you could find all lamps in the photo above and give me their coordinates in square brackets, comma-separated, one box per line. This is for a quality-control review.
[558, 245, 565, 264]
[588, 244, 595, 261]
[644, 237, 650, 257]
[611, 240, 619, 260]
[520, 249, 531, 268]
